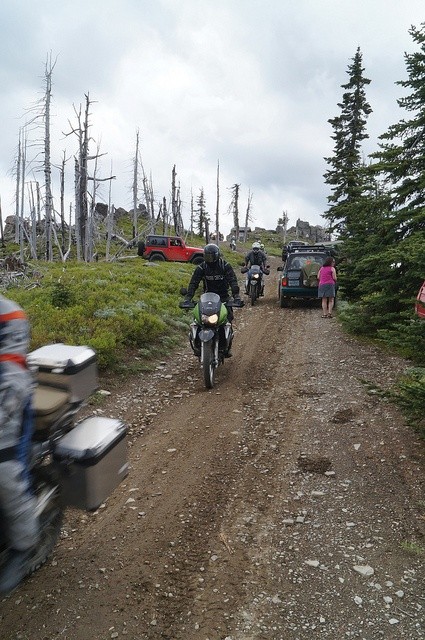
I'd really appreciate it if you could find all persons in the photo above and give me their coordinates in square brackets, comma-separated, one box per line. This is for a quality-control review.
[245, 242, 269, 294]
[184, 244, 240, 322]
[0, 290, 42, 589]
[317, 258, 336, 318]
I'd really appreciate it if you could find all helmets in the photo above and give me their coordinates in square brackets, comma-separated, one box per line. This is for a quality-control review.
[258, 240, 261, 244]
[261, 244, 264, 248]
[203, 244, 219, 264]
[252, 242, 261, 248]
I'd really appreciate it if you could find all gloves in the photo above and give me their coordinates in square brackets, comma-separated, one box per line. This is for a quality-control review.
[232, 294, 242, 304]
[184, 295, 193, 312]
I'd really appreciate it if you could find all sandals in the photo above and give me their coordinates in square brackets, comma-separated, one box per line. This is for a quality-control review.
[328, 313, 334, 318]
[320, 314, 327, 318]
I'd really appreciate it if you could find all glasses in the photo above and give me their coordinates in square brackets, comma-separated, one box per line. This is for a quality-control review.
[332, 261, 336, 263]
[253, 249, 258, 251]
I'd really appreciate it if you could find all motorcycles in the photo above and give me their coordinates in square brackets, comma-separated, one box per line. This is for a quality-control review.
[237, 263, 267, 308]
[229, 236, 239, 252]
[0, 329, 130, 621]
[174, 300, 246, 391]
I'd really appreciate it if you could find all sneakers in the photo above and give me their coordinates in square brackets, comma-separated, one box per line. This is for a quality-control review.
[225, 350, 232, 358]
[0, 546, 36, 572]
[194, 347, 201, 356]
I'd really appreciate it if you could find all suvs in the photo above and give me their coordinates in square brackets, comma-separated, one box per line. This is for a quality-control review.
[138, 234, 208, 264]
[283, 238, 347, 250]
[277, 253, 339, 310]
